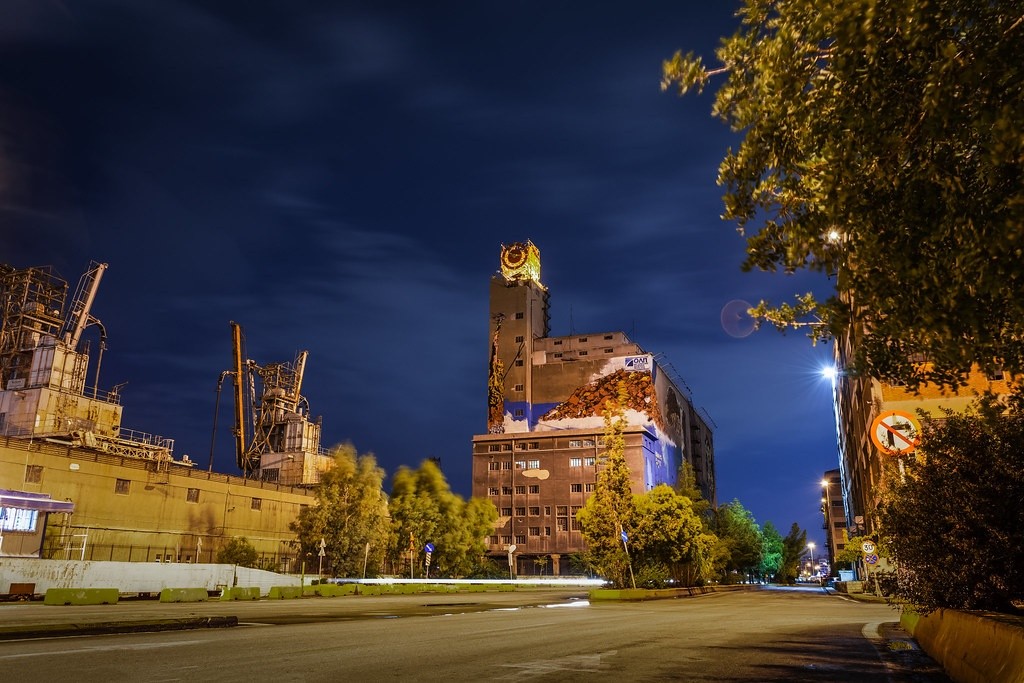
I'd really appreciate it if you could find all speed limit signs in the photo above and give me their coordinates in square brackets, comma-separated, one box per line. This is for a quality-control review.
[862, 540, 876, 554]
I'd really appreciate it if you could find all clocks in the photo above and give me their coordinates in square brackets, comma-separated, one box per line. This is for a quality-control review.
[502, 243, 528, 269]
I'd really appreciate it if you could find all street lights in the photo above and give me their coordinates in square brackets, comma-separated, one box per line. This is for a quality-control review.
[808, 542, 815, 576]
